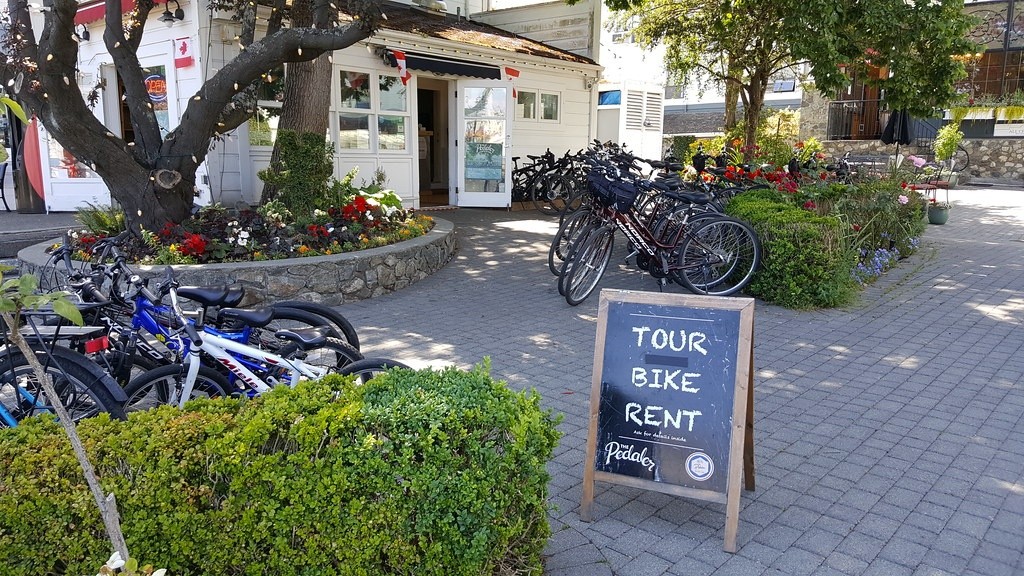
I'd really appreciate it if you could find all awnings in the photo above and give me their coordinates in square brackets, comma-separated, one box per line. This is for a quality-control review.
[74, 0, 168, 27]
[384, 45, 502, 80]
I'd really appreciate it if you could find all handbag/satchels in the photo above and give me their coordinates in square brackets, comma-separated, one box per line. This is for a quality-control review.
[586, 170, 639, 214]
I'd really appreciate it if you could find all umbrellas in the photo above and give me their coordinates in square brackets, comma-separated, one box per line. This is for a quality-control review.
[881, 109, 915, 160]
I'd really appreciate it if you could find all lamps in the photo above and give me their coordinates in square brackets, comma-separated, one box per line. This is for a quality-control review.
[72, 23, 90, 42]
[157, 0, 184, 28]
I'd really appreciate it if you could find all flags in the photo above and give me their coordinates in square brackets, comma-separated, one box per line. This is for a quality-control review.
[175, 36, 192, 68]
[345, 72, 366, 89]
[505, 67, 520, 98]
[393, 50, 412, 86]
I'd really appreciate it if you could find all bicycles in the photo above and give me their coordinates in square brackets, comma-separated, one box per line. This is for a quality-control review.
[0, 231, 417, 428]
[512, 139, 762, 307]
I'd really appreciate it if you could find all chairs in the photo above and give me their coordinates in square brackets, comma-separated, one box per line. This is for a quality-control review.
[0, 163, 11, 212]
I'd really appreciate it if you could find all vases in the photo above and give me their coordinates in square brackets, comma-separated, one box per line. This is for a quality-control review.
[928, 205, 950, 225]
